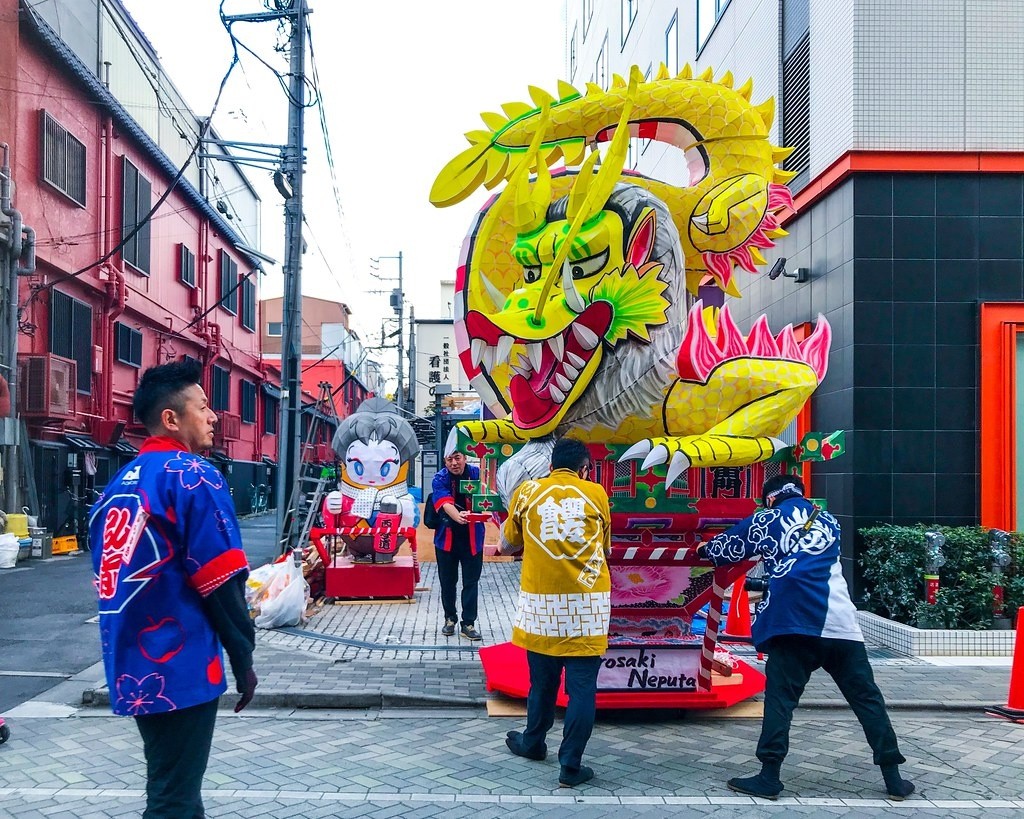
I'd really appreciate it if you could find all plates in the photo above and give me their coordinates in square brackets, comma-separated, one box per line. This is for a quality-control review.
[466, 514, 491, 522]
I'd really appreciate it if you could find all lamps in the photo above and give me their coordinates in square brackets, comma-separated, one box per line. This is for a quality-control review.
[309, 465, 313, 474]
[266, 468, 271, 476]
[228, 465, 233, 474]
[68, 453, 78, 468]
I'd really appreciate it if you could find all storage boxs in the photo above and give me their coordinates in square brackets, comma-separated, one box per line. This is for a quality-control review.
[29, 533, 53, 559]
[52, 535, 79, 556]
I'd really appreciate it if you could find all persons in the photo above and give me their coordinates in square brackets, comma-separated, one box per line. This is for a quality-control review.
[86, 360, 259, 819]
[696, 474, 915, 801]
[434, 446, 486, 641]
[494, 438, 612, 786]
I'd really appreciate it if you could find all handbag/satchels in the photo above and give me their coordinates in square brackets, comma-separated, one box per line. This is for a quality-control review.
[244, 555, 308, 629]
[424, 493, 441, 529]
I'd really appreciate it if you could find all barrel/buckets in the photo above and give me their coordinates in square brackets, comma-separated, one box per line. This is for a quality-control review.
[0, 513, 32, 568]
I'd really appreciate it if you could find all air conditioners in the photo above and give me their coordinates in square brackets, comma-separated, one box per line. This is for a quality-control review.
[216, 413, 239, 438]
[24, 355, 71, 413]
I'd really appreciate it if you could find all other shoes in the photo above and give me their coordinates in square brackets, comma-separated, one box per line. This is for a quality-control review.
[558, 766, 593, 787]
[727, 775, 780, 800]
[506, 731, 546, 761]
[887, 780, 915, 799]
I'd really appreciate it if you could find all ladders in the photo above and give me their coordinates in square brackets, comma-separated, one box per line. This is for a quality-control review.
[271, 380, 341, 561]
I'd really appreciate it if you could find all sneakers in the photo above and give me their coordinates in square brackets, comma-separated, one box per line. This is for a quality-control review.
[442, 616, 455, 635]
[461, 624, 482, 640]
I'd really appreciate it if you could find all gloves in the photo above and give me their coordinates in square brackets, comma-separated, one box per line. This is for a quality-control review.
[233, 660, 257, 713]
